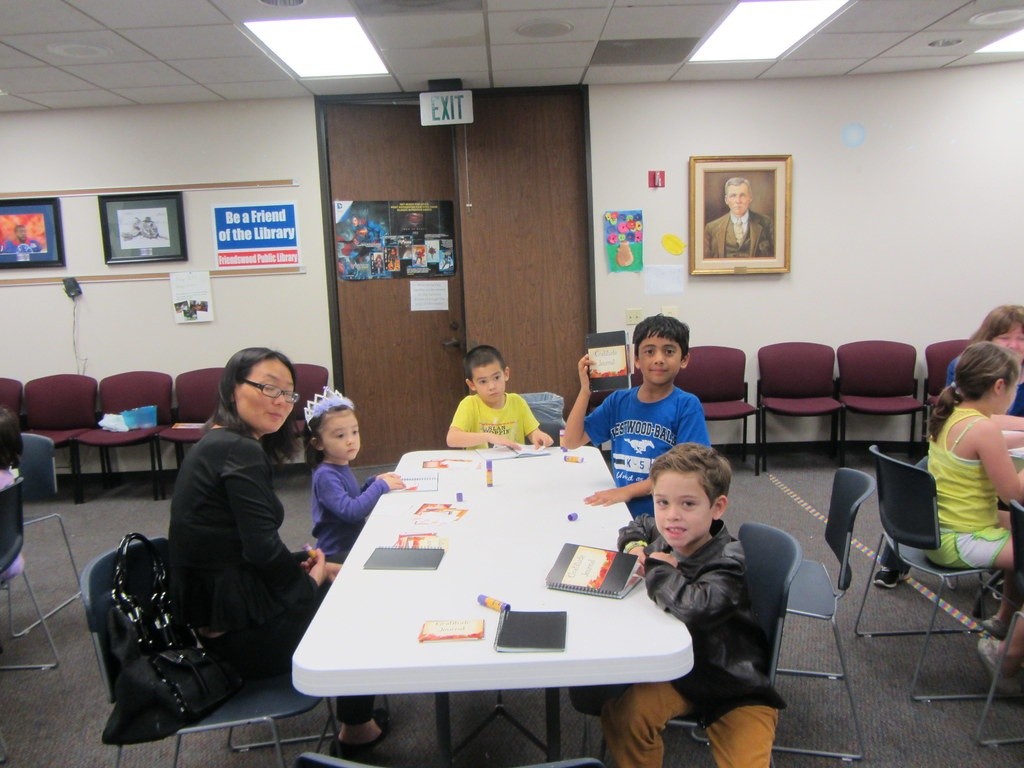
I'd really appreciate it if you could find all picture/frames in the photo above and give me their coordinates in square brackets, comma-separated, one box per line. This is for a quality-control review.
[97, 191, 187, 265]
[689, 155, 793, 275]
[0, 197, 66, 269]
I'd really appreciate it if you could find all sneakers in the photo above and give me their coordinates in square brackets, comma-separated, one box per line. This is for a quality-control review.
[873, 565, 911, 588]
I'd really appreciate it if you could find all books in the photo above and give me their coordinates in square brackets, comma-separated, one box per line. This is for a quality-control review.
[585, 330, 632, 392]
[545, 543, 642, 599]
[388, 470, 438, 491]
[494, 610, 567, 652]
[475, 444, 552, 460]
[363, 547, 444, 570]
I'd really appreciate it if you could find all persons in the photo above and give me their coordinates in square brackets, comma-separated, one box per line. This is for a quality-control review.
[446, 345, 554, 451]
[927, 341, 1024, 698]
[167, 347, 390, 759]
[302, 387, 406, 564]
[0, 224, 41, 253]
[0, 404, 25, 581]
[601, 441, 787, 768]
[562, 315, 711, 520]
[945, 305, 1024, 418]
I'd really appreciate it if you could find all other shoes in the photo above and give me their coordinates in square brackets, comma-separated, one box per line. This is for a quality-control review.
[981, 616, 1008, 639]
[330, 708, 388, 759]
[978, 639, 1020, 694]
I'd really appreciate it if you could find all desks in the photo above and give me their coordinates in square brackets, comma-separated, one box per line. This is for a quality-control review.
[290, 446, 695, 763]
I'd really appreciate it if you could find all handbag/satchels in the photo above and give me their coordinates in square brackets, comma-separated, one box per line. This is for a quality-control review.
[101, 529, 242, 745]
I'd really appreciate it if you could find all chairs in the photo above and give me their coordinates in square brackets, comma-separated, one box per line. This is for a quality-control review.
[0, 364, 344, 767]
[569, 334, 1024, 767]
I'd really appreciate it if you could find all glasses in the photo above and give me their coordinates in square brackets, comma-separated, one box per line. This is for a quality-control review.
[241, 377, 300, 403]
